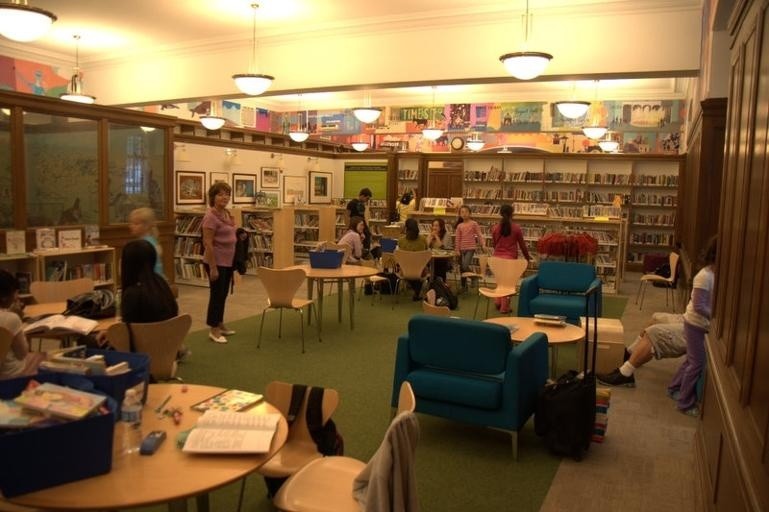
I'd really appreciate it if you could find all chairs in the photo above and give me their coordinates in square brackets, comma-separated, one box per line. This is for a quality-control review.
[635, 251, 680, 312]
[517, 259, 602, 325]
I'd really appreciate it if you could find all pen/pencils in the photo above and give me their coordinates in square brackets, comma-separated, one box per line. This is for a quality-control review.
[155, 395, 172, 413]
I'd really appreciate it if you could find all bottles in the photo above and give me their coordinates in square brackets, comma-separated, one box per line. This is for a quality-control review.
[122, 389, 143, 453]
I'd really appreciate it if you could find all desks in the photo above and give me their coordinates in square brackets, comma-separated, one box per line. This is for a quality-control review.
[481, 318, 585, 380]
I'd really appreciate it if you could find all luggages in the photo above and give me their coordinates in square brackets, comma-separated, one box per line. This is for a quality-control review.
[534, 284, 601, 463]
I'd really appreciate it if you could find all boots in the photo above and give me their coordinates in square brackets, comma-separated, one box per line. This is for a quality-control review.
[364, 284, 373, 295]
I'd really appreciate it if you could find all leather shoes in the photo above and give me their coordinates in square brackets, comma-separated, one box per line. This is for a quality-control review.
[209, 331, 228, 344]
[218, 329, 236, 335]
[414, 296, 423, 301]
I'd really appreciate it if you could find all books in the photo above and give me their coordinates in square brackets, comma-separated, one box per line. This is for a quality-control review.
[2, 223, 116, 305]
[0, 381, 108, 427]
[463, 167, 678, 227]
[41, 341, 132, 383]
[291, 209, 325, 268]
[181, 410, 286, 456]
[190, 389, 264, 412]
[23, 314, 95, 335]
[174, 215, 230, 286]
[242, 213, 275, 275]
[325, 195, 388, 248]
[397, 183, 683, 280]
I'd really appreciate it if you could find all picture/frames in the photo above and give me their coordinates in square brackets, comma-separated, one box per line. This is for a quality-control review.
[34, 225, 57, 250]
[2, 229, 27, 254]
[55, 226, 85, 248]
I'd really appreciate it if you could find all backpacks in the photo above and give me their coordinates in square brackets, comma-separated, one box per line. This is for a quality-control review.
[263, 384, 344, 498]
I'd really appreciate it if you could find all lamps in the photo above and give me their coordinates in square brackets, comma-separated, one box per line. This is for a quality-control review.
[0, 0, 58, 42]
[60, 35, 96, 104]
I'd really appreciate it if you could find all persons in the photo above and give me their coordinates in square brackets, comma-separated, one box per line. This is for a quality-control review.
[600, 308, 686, 390]
[629, 103, 661, 127]
[670, 225, 719, 419]
[337, 185, 483, 309]
[96, 239, 182, 382]
[0, 268, 45, 378]
[127, 207, 166, 280]
[200, 183, 249, 344]
[489, 205, 530, 313]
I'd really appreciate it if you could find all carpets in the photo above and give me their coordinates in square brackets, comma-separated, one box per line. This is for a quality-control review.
[159, 280, 630, 512]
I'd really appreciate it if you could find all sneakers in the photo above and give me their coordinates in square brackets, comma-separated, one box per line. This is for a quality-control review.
[457, 288, 468, 295]
[666, 386, 682, 400]
[624, 347, 632, 363]
[681, 404, 700, 417]
[596, 367, 636, 387]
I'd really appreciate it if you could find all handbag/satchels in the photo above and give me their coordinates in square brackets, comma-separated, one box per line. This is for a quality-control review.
[380, 272, 399, 294]
[66, 289, 117, 320]
[388, 209, 400, 224]
